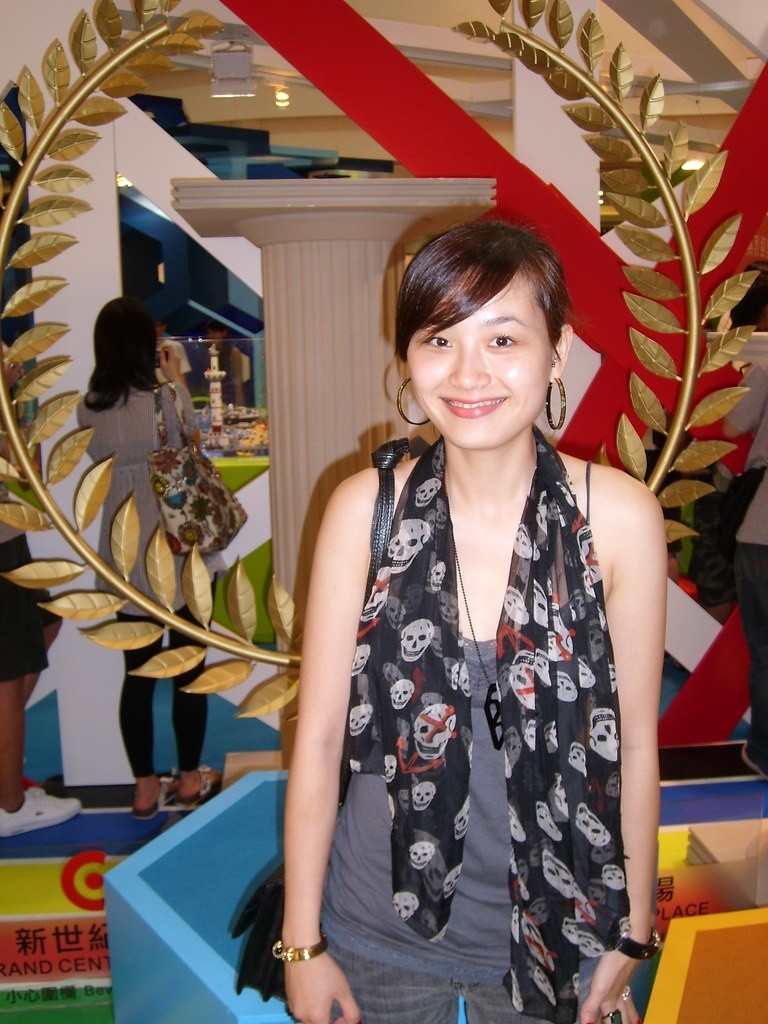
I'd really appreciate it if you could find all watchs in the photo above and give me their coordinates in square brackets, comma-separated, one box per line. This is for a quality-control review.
[607, 916, 660, 959]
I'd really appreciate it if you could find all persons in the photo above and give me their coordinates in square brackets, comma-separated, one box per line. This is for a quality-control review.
[206, 320, 244, 406]
[154, 314, 190, 421]
[0, 343, 81, 836]
[78, 299, 222, 820]
[272, 222, 666, 1024]
[693, 261, 768, 781]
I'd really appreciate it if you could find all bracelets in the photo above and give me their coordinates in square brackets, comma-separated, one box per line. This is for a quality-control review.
[272, 934, 327, 962]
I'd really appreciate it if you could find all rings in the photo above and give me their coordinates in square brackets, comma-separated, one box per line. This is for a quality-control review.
[622, 986, 632, 1000]
[9, 363, 16, 368]
[603, 1009, 622, 1024]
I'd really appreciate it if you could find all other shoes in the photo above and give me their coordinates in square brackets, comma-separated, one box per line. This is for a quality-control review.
[742, 742, 768, 780]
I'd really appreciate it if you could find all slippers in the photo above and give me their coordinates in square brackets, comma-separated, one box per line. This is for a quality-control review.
[175, 765, 222, 808]
[132, 777, 179, 820]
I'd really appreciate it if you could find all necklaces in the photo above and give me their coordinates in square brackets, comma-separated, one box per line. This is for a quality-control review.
[452, 537, 504, 750]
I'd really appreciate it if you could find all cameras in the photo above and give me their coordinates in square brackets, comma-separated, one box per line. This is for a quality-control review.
[155, 350, 168, 368]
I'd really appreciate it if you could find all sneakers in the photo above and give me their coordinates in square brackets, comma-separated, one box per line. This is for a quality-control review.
[0, 788, 82, 836]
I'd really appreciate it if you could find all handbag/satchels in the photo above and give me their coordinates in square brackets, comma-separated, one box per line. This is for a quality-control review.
[146, 383, 247, 555]
[231, 861, 287, 1000]
[716, 466, 765, 563]
